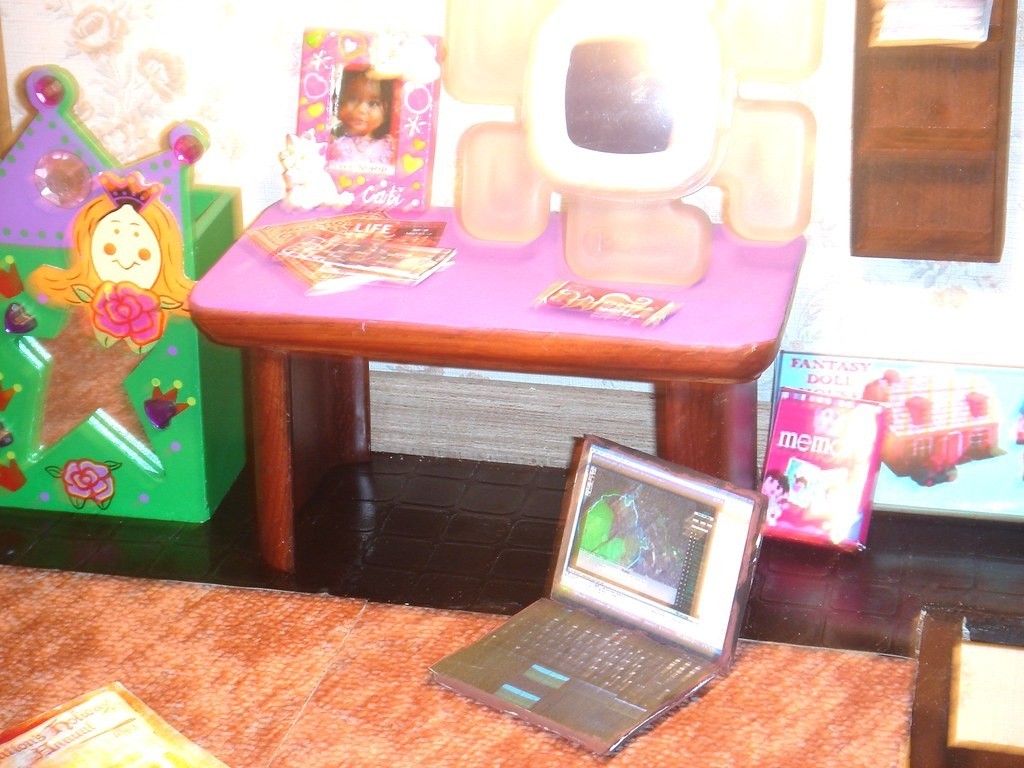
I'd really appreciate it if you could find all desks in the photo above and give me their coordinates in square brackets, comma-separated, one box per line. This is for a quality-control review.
[187, 197, 809, 574]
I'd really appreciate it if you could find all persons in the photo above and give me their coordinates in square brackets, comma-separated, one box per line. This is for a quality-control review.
[328, 62, 397, 172]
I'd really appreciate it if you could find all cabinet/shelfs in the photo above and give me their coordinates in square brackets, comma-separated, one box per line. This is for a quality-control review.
[850, 1, 1018, 262]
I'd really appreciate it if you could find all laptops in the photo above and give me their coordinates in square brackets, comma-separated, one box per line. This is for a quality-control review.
[427, 430, 766, 759]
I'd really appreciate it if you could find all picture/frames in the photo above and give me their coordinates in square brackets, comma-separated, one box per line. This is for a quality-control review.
[278, 26, 448, 216]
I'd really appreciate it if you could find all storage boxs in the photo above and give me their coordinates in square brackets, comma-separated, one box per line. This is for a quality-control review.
[1, 66, 245, 523]
[755, 385, 891, 553]
[768, 348, 1022, 525]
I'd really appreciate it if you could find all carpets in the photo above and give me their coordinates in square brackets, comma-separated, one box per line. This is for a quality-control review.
[0, 561, 917, 768]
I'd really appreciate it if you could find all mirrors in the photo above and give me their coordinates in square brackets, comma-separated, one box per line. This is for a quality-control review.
[523, 6, 739, 197]
